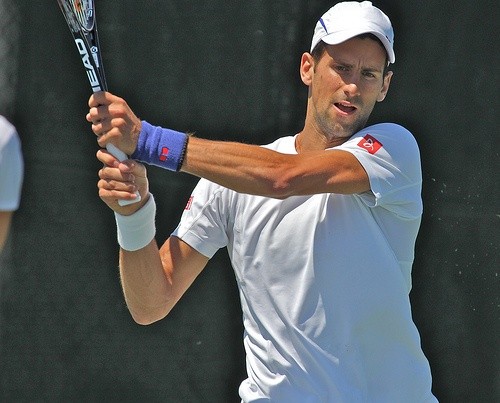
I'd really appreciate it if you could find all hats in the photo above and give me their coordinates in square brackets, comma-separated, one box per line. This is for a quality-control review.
[310, 1, 395, 67]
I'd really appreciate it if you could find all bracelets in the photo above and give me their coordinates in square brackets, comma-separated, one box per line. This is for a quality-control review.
[115, 192, 156, 251]
[132, 121, 188, 173]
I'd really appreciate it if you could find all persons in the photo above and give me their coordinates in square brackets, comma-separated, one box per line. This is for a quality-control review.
[0, 114, 23, 246]
[87, 1, 438, 403]
